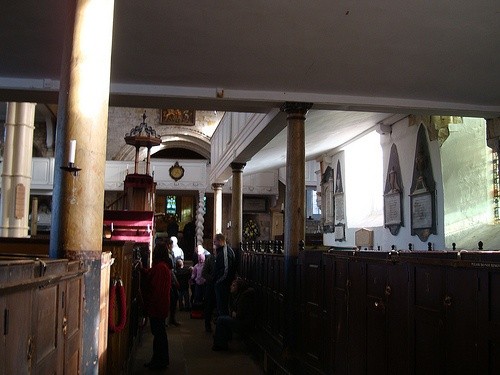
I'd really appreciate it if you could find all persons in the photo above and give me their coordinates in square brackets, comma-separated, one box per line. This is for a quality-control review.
[134, 244, 174, 370]
[211, 281, 254, 351]
[153, 234, 237, 334]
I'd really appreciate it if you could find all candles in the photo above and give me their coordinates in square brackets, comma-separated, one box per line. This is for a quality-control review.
[111, 222, 114, 230]
[68, 139, 76, 164]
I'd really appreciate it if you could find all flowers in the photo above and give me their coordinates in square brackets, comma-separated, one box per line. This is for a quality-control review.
[243, 218, 261, 242]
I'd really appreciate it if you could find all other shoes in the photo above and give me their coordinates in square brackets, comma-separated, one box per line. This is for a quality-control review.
[168, 319, 180, 326]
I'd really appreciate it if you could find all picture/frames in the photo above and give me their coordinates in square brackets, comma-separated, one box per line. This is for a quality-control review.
[158, 108, 196, 126]
